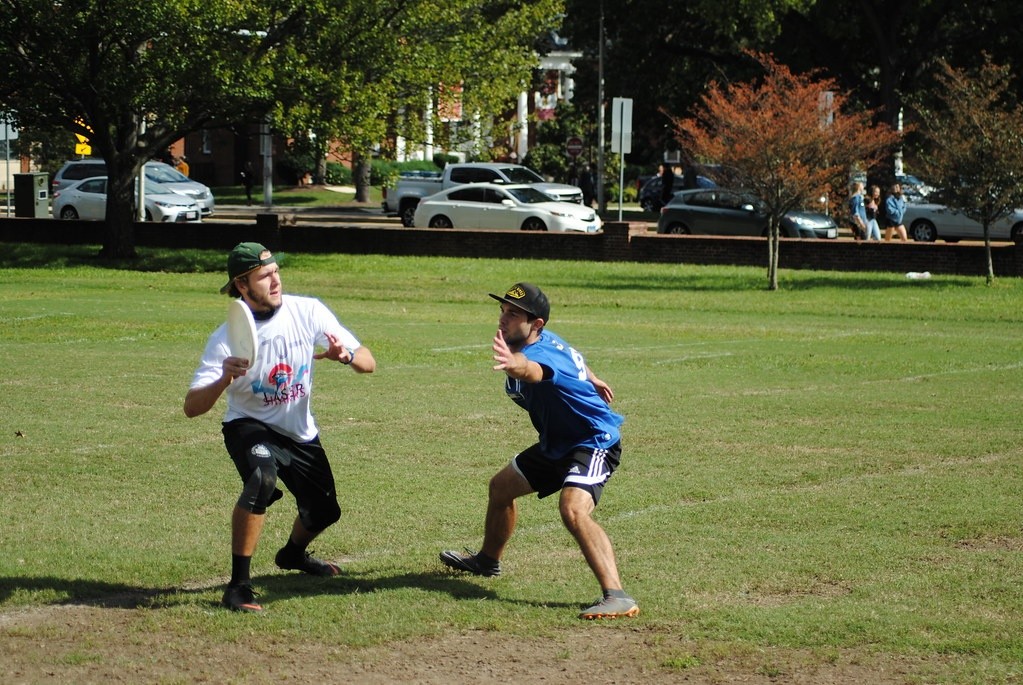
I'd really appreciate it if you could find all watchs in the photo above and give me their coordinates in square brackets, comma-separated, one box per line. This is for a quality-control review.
[341, 347, 355, 365]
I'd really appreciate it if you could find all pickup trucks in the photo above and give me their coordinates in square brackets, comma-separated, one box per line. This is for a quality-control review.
[381, 161, 585, 229]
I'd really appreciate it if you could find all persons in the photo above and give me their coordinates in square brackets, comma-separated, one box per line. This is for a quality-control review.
[566, 161, 598, 207]
[241, 162, 256, 205]
[183, 242, 377, 612]
[440, 283, 639, 621]
[847, 179, 908, 242]
[656, 164, 674, 207]
[680, 157, 698, 188]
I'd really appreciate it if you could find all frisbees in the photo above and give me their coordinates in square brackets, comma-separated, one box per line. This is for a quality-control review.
[227, 299, 259, 373]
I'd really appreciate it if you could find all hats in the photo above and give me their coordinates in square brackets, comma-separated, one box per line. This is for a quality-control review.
[221, 240, 276, 294]
[489, 281, 549, 325]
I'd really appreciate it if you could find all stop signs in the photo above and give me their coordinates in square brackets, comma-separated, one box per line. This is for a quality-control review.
[567, 137, 583, 156]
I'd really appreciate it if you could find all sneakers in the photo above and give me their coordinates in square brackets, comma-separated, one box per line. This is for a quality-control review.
[223, 584, 261, 613]
[274, 551, 343, 577]
[440, 546, 500, 576]
[580, 592, 640, 621]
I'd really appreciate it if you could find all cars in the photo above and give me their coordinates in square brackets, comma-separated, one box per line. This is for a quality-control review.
[412, 182, 602, 234]
[51, 174, 202, 223]
[901, 189, 1023, 245]
[51, 157, 215, 218]
[635, 159, 743, 215]
[894, 171, 922, 197]
[656, 186, 840, 239]
